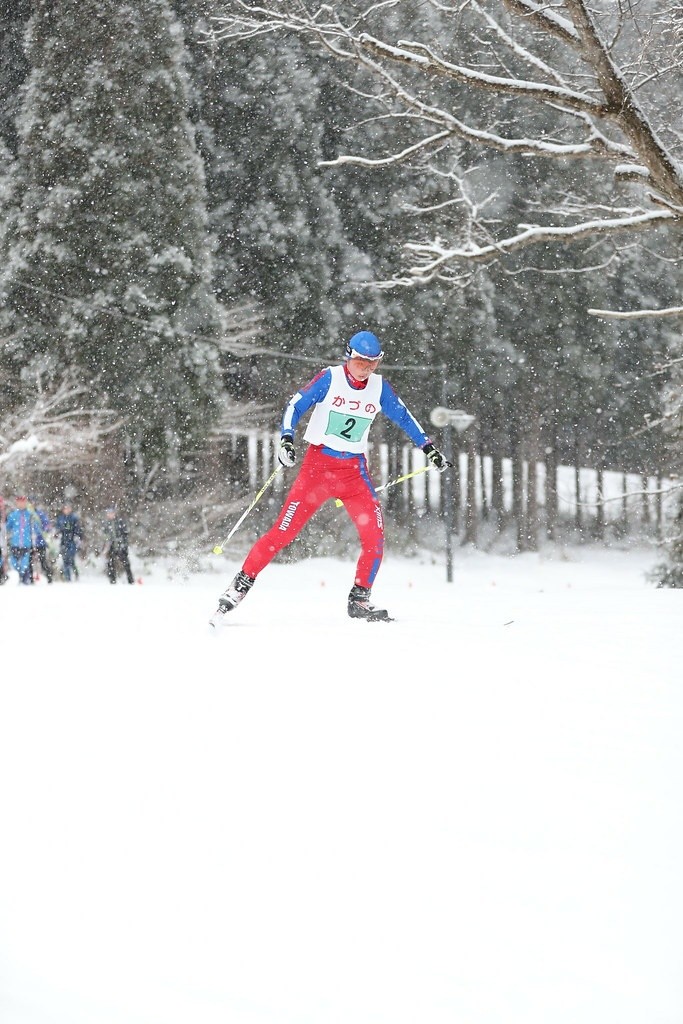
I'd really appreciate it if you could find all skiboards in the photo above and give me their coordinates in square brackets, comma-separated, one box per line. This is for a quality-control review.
[210, 607, 405, 632]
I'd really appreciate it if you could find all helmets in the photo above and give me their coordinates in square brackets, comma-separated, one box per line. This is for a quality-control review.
[347, 332, 384, 359]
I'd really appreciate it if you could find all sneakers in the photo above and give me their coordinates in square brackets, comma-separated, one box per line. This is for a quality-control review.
[218, 572, 254, 610]
[347, 586, 389, 621]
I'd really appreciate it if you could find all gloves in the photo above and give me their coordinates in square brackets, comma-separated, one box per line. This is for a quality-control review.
[278, 434, 296, 468]
[422, 444, 449, 471]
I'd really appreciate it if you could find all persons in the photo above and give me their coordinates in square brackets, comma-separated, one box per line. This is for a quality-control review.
[0, 495, 86, 587]
[96, 506, 136, 585]
[218, 330, 449, 622]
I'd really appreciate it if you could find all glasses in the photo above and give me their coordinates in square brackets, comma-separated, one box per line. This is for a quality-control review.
[351, 354, 385, 369]
